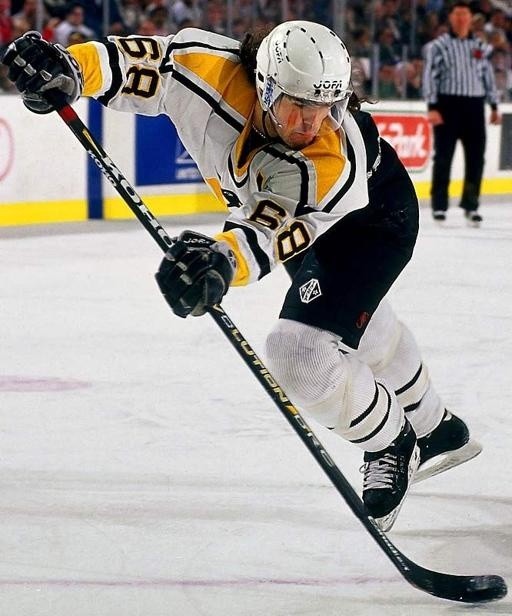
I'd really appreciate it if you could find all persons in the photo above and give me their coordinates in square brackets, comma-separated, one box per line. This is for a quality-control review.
[421, 5, 501, 223]
[1, 1, 512, 103]
[3, 20, 482, 534]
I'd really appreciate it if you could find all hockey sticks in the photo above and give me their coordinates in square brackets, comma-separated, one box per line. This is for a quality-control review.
[21, 32, 507, 603]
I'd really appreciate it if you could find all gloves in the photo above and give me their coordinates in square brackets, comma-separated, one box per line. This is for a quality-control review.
[155, 231, 238, 316]
[4, 33, 84, 116]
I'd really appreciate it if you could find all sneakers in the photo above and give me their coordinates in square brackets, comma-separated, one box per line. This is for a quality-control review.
[433, 209, 446, 221]
[359, 409, 468, 519]
[464, 208, 482, 221]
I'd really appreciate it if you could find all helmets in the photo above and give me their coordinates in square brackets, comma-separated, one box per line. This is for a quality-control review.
[258, 20, 353, 138]
